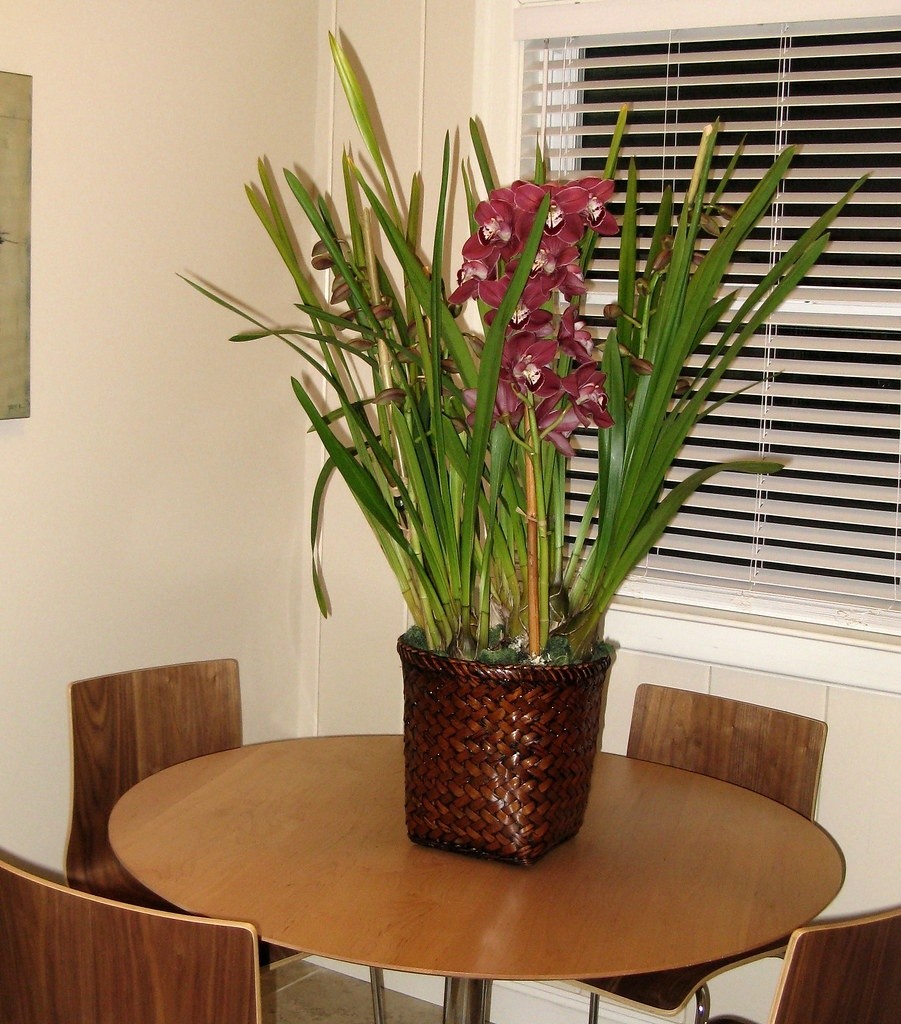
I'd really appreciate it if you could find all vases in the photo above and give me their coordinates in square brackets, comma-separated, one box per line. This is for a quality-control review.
[395, 626, 611, 866]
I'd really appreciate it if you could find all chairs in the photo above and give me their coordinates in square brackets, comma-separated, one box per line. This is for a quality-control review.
[0, 861, 262, 1024]
[476, 682, 828, 1024]
[703, 907, 901, 1023]
[62, 659, 386, 1024]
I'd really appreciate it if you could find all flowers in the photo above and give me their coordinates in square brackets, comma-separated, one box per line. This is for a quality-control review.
[171, 20, 874, 666]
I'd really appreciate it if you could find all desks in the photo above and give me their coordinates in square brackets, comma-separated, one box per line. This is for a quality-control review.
[107, 734, 844, 1024]
